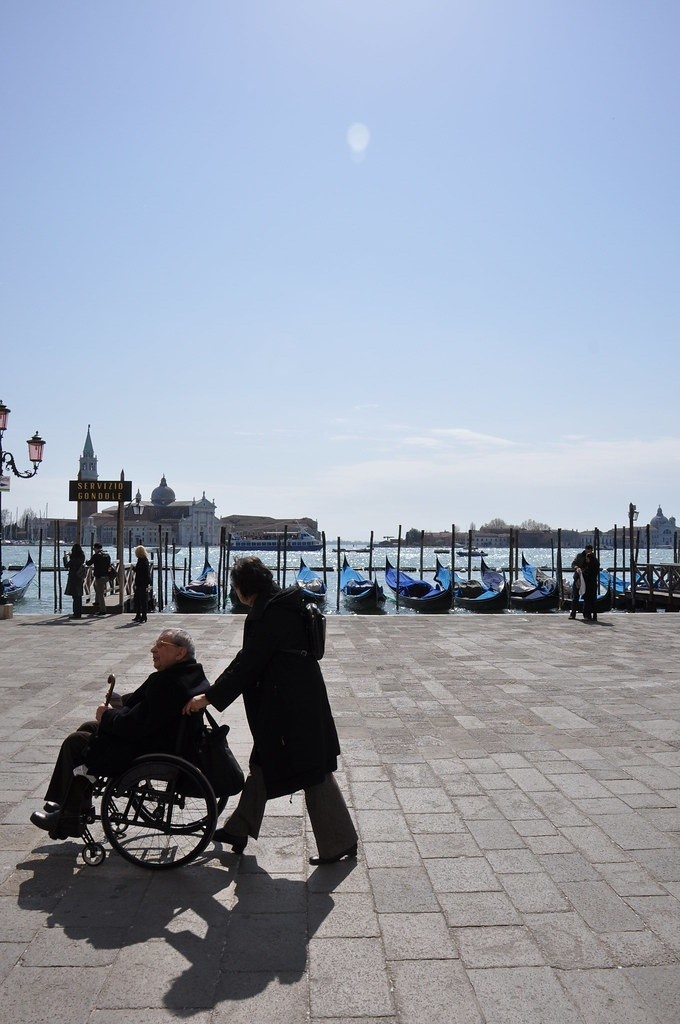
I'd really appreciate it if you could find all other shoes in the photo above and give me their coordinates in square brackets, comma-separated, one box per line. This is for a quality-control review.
[92, 602, 100, 606]
[69, 612, 81, 619]
[95, 611, 105, 614]
[584, 618, 588, 621]
[568, 615, 575, 619]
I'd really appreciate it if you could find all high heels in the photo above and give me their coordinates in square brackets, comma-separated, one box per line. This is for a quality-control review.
[132, 615, 141, 621]
[309, 844, 357, 865]
[137, 616, 147, 622]
[201, 826, 247, 854]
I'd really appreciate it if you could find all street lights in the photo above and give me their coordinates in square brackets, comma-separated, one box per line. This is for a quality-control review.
[0, 398, 47, 606]
[125, 497, 145, 516]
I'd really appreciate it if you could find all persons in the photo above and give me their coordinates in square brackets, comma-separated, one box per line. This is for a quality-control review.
[581, 553, 600, 621]
[182, 556, 358, 863]
[86, 543, 111, 615]
[30, 627, 211, 838]
[568, 544, 594, 619]
[131, 545, 152, 623]
[63, 543, 85, 619]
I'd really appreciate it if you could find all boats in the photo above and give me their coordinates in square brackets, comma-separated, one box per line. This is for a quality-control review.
[225, 520, 323, 551]
[521, 552, 612, 613]
[434, 550, 450, 554]
[146, 546, 181, 554]
[294, 556, 327, 601]
[169, 557, 225, 612]
[433, 554, 509, 611]
[480, 557, 559, 610]
[0, 550, 38, 600]
[596, 569, 631, 592]
[385, 555, 452, 612]
[332, 548, 371, 552]
[340, 553, 384, 609]
[456, 550, 489, 557]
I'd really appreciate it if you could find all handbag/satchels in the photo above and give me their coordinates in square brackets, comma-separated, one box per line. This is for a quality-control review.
[175, 708, 245, 798]
[579, 569, 586, 595]
[105, 567, 120, 581]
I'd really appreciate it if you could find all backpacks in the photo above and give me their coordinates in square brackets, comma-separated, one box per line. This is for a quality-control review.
[279, 602, 326, 660]
[76, 565, 87, 578]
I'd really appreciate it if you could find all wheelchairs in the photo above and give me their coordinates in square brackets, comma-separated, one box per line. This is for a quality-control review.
[45, 707, 229, 870]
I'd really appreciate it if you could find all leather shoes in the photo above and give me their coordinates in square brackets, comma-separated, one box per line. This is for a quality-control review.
[44, 802, 95, 824]
[30, 811, 82, 837]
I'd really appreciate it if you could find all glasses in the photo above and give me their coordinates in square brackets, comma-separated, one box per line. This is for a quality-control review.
[156, 640, 178, 648]
